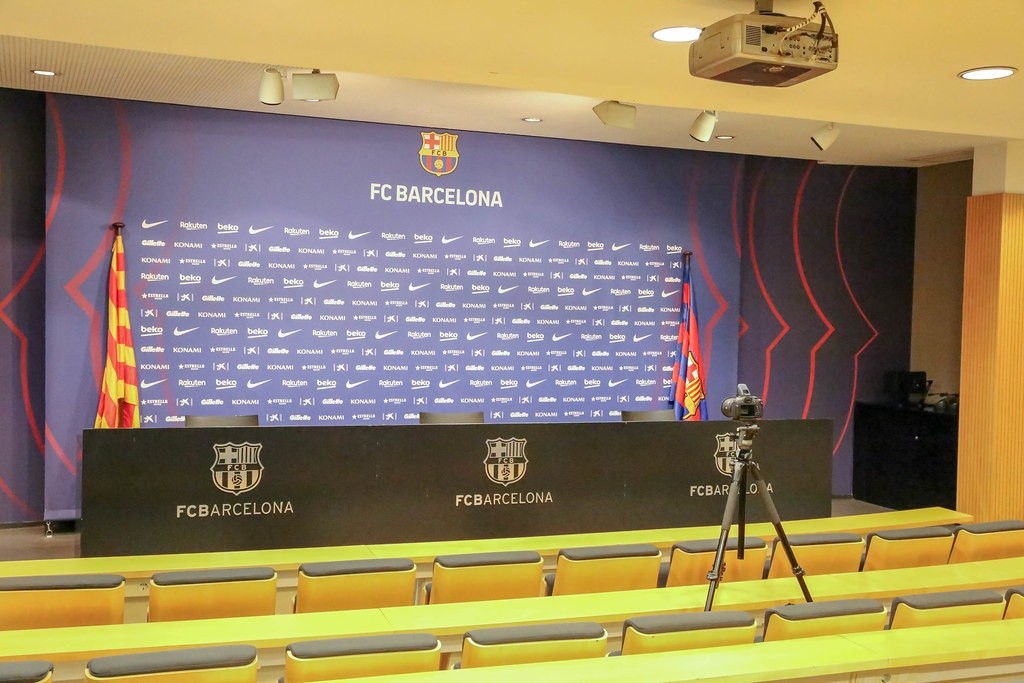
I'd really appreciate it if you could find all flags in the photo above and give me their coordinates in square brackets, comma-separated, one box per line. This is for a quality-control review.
[92, 222, 144, 429]
[667, 252, 711, 420]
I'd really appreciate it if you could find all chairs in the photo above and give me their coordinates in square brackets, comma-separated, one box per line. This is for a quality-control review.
[762, 533, 865, 577]
[277, 633, 442, 683]
[292, 558, 417, 614]
[540, 543, 662, 597]
[449, 622, 608, 670]
[0, 574, 126, 631]
[83, 644, 258, 683]
[0, 661, 55, 683]
[605, 610, 757, 657]
[948, 520, 1024, 564]
[621, 409, 675, 421]
[419, 412, 484, 424]
[882, 589, 1008, 630]
[1002, 585, 1024, 619]
[754, 598, 888, 642]
[859, 526, 955, 572]
[657, 536, 768, 588]
[420, 551, 544, 605]
[147, 566, 277, 622]
[185, 415, 258, 427]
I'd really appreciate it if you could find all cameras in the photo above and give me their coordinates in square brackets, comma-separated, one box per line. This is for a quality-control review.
[721, 383, 764, 425]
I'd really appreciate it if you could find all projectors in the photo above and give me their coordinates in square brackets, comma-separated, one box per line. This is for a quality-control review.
[689, 0, 840, 88]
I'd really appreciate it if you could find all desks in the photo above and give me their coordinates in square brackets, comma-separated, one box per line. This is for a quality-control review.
[0, 506, 1024, 683]
[851, 400, 959, 509]
[80, 418, 834, 558]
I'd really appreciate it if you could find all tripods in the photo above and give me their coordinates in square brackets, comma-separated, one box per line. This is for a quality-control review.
[704, 425, 814, 613]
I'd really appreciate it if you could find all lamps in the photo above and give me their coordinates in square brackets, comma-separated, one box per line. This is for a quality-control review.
[592, 100, 637, 130]
[689, 110, 718, 143]
[290, 69, 340, 101]
[810, 122, 841, 151]
[258, 68, 287, 105]
